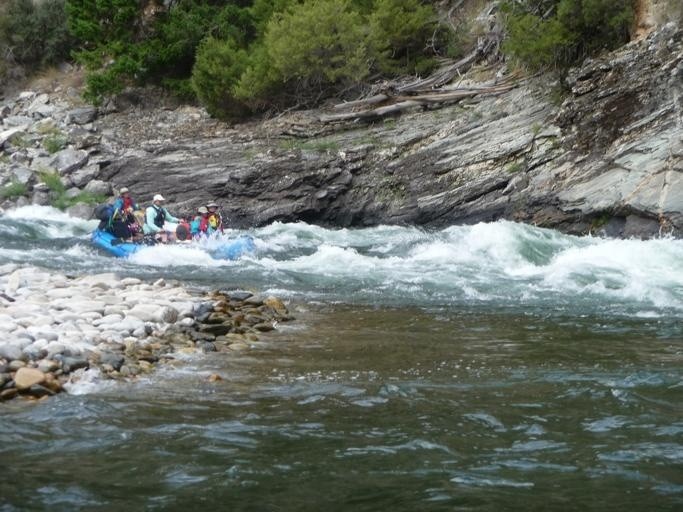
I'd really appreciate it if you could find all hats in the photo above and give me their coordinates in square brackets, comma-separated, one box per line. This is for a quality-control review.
[153, 194, 165, 201]
[119, 187, 129, 194]
[197, 201, 219, 213]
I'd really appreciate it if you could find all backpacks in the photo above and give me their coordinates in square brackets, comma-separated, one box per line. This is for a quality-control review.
[176, 222, 192, 240]
[92, 204, 113, 219]
[209, 215, 218, 229]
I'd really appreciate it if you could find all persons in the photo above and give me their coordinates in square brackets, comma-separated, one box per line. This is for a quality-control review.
[98, 186, 224, 244]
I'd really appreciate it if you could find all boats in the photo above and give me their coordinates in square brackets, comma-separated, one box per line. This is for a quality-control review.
[92, 227, 255, 259]
